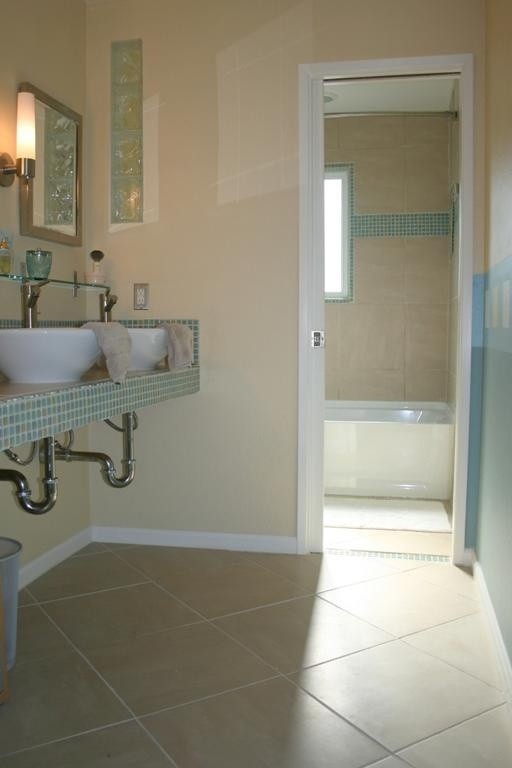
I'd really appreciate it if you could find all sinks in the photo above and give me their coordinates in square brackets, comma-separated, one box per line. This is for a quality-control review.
[128, 326, 169, 371]
[1, 326, 101, 383]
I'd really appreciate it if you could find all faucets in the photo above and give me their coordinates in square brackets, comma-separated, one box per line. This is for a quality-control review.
[99, 288, 118, 322]
[21, 281, 52, 327]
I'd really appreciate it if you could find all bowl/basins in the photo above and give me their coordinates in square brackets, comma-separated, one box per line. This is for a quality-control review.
[83, 272, 108, 286]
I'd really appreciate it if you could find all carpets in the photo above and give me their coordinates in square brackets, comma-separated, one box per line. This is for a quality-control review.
[323, 494, 451, 535]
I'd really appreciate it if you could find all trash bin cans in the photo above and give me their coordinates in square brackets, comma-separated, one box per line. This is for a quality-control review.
[0, 536, 22, 671]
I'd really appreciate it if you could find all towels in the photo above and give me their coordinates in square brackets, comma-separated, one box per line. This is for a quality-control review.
[155, 322, 195, 372]
[80, 322, 131, 386]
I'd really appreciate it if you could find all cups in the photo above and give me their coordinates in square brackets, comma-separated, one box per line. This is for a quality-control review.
[0, 252, 12, 275]
[26, 250, 53, 280]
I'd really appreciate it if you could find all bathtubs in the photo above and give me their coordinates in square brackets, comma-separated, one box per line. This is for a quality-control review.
[323, 398, 454, 502]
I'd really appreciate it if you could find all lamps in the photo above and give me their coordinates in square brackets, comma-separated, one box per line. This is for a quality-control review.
[1, 90, 36, 186]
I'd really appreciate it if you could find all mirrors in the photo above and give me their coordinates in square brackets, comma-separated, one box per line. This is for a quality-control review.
[16, 81, 83, 248]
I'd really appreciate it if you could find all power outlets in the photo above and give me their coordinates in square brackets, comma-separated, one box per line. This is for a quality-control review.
[132, 282, 150, 311]
